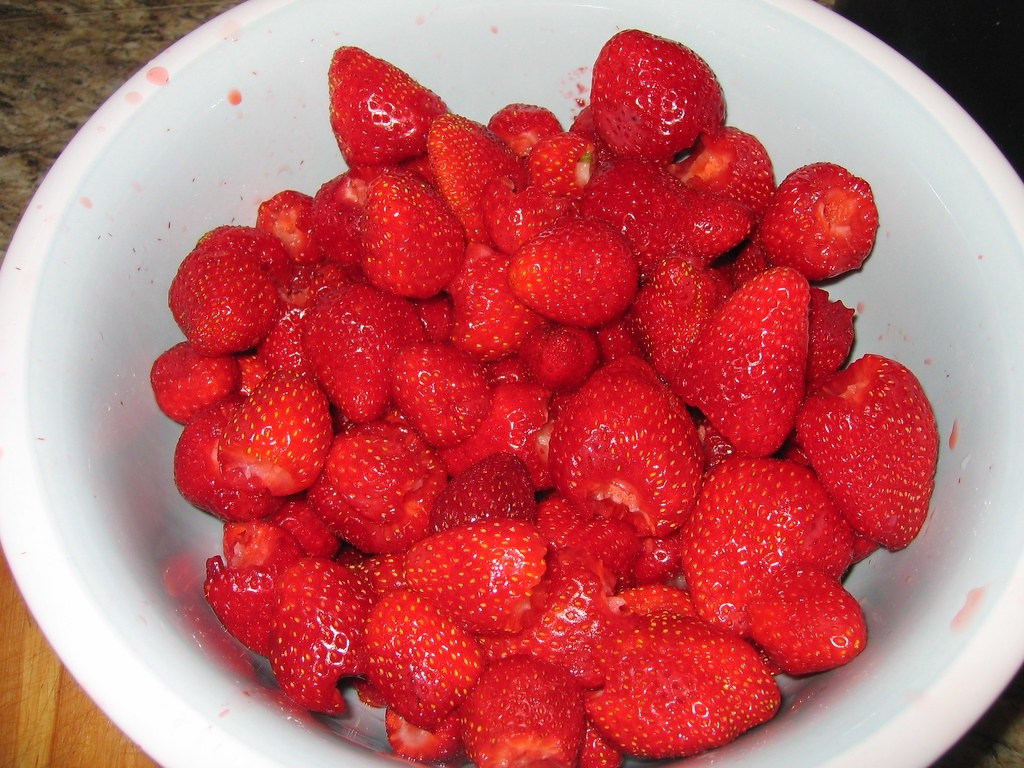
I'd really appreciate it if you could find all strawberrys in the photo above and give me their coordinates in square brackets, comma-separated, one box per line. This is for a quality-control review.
[149, 29, 938, 768]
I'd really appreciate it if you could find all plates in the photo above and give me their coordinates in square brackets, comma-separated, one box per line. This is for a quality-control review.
[2, 1, 1022, 768]
